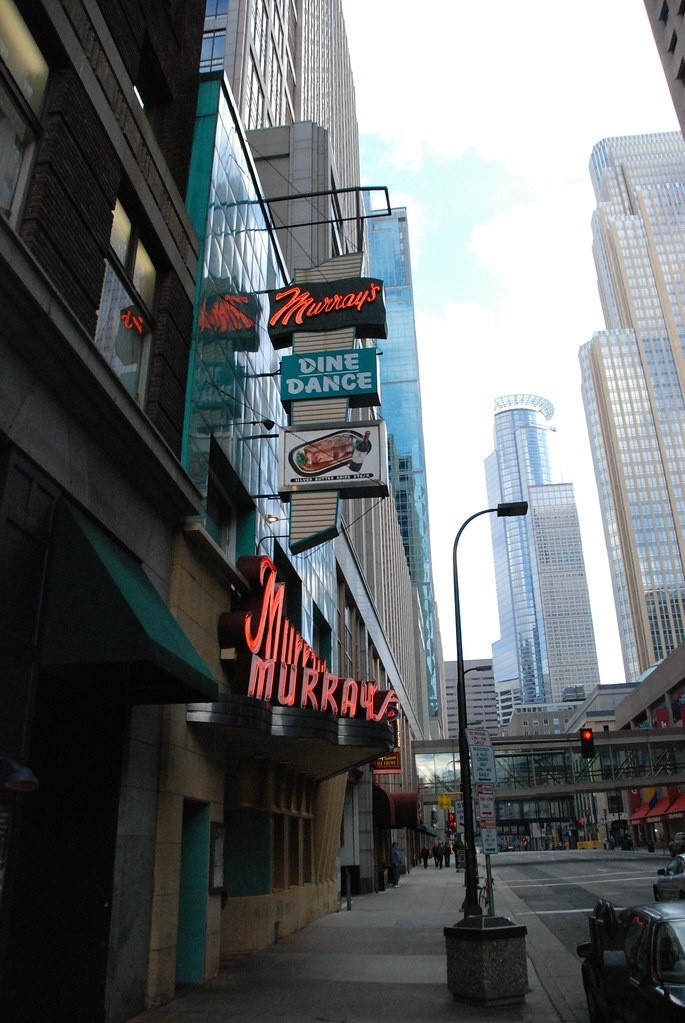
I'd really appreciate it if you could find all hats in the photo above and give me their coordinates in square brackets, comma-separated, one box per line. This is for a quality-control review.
[393, 841, 399, 845]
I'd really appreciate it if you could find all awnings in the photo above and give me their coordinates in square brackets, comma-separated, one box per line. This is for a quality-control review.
[44, 497, 220, 704]
[628, 791, 685, 824]
[421, 824, 438, 837]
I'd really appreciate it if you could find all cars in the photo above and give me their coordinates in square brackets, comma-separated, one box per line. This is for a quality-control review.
[668, 832, 685, 856]
[653, 853, 685, 902]
[576, 900, 685, 1023]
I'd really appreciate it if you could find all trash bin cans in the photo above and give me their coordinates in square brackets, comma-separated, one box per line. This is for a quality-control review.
[340, 864, 360, 897]
[647, 841, 654, 852]
[443, 915, 534, 1008]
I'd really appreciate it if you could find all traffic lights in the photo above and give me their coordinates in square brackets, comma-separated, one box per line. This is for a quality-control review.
[579, 727, 595, 758]
[577, 818, 584, 825]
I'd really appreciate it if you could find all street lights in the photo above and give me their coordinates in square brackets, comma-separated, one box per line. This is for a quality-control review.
[453, 500, 528, 915]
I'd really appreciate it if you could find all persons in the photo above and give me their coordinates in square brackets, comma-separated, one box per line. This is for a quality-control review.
[421, 842, 451, 869]
[391, 841, 402, 888]
[659, 834, 665, 848]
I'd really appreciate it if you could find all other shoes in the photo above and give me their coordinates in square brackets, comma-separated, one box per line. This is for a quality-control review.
[395, 885, 400, 888]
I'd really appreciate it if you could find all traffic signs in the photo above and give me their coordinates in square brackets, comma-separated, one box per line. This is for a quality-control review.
[478, 794, 496, 817]
[469, 745, 497, 785]
[482, 829, 498, 854]
